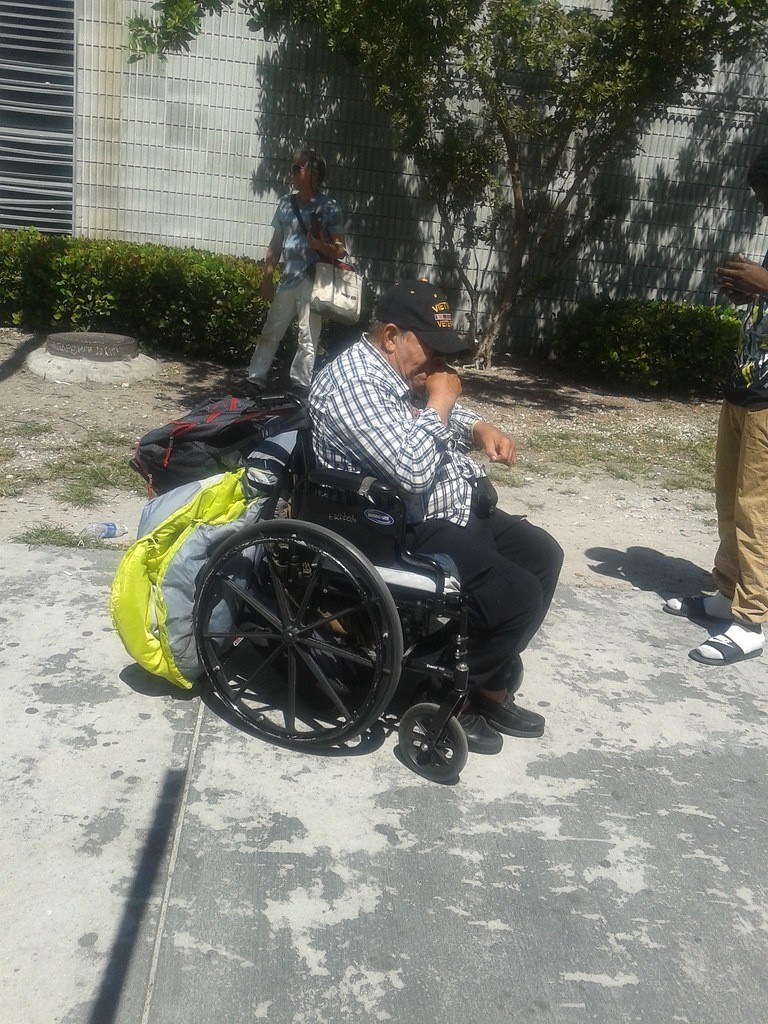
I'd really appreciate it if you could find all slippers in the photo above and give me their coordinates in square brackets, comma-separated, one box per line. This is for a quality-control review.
[663, 595, 736, 622]
[688, 633, 763, 666]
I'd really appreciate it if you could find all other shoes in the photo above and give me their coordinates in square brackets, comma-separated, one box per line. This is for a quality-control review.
[240, 381, 261, 396]
[291, 384, 309, 398]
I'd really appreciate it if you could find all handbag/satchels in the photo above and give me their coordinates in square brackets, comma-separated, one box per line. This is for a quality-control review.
[130, 393, 314, 497]
[311, 242, 365, 326]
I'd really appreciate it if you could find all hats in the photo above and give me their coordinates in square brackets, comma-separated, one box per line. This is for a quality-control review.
[374, 278, 471, 357]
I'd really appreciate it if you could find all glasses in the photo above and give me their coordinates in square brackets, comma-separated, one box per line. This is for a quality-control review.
[291, 163, 319, 175]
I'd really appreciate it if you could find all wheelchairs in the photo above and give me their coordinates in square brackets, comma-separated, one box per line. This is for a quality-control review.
[192, 385, 471, 783]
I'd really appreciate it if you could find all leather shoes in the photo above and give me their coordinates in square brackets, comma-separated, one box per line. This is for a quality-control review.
[410, 682, 503, 755]
[480, 693, 546, 738]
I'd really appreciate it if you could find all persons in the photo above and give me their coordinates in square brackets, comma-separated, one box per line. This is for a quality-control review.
[238, 148, 345, 398]
[662, 144, 768, 667]
[308, 278, 563, 753]
